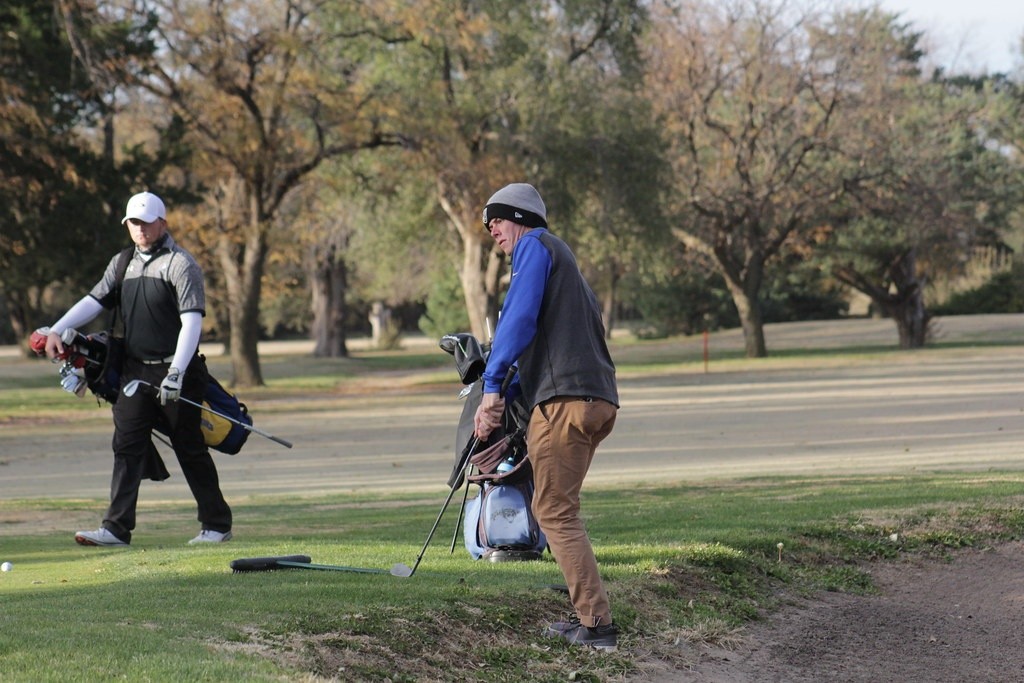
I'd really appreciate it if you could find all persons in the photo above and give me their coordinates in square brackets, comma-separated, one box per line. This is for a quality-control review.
[44, 191, 233, 546]
[476, 181, 622, 652]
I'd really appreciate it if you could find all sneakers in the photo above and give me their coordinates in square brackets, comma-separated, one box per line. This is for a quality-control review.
[73, 528, 129, 547]
[187, 529, 231, 547]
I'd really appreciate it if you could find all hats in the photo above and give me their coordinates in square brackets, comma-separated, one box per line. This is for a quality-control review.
[483, 182, 550, 235]
[120, 191, 168, 223]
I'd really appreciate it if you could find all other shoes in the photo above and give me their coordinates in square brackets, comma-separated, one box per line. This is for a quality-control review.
[544, 582, 572, 596]
[548, 621, 620, 653]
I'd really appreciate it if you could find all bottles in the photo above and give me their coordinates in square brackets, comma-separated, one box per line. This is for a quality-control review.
[496, 457, 514, 477]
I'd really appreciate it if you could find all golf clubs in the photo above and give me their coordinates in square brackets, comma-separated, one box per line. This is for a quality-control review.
[52, 349, 92, 398]
[390, 365, 519, 577]
[123, 379, 293, 450]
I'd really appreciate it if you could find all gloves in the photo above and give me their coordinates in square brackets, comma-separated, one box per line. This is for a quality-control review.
[156, 368, 186, 406]
